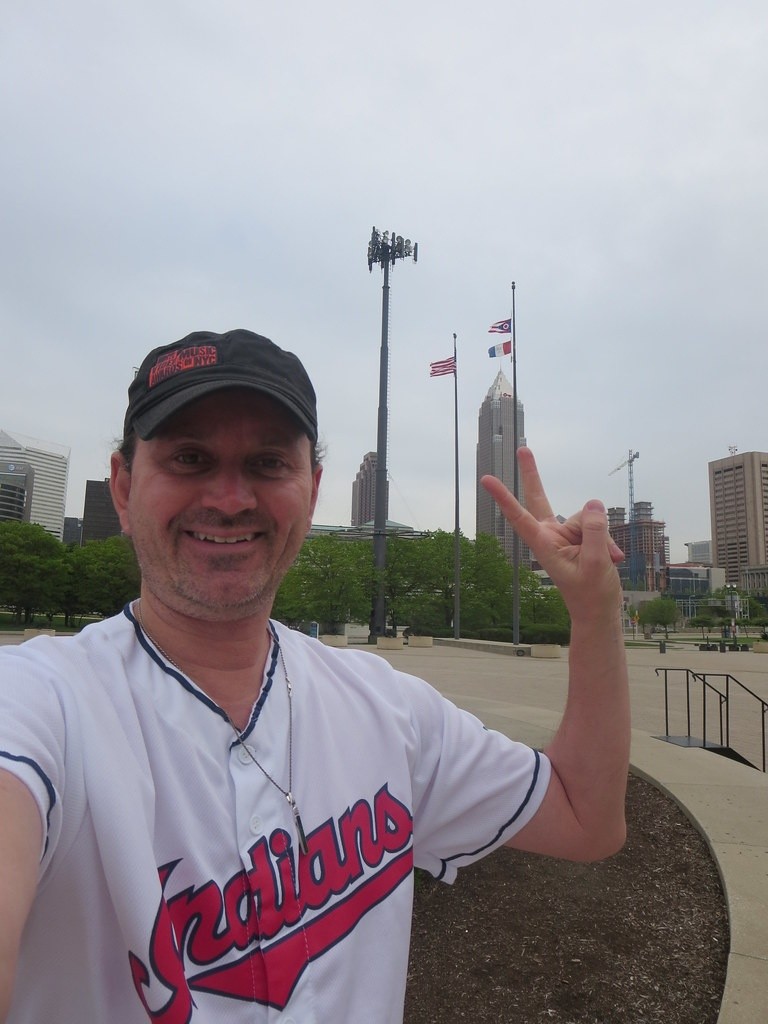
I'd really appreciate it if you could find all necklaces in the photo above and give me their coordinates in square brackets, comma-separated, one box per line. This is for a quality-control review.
[137, 599, 308, 855]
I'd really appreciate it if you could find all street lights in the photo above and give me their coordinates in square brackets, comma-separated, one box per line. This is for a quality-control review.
[726, 584, 740, 651]
[366, 226, 420, 648]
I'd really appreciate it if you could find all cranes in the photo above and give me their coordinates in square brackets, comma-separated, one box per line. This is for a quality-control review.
[608, 451, 642, 592]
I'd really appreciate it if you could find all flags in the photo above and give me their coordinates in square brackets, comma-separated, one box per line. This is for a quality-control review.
[430, 357, 454, 376]
[487, 318, 511, 333]
[488, 341, 511, 358]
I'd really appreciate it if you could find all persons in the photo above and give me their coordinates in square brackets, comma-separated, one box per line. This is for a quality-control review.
[0, 329, 632, 1024]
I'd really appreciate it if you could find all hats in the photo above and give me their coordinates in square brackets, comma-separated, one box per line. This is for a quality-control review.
[123, 328, 318, 443]
